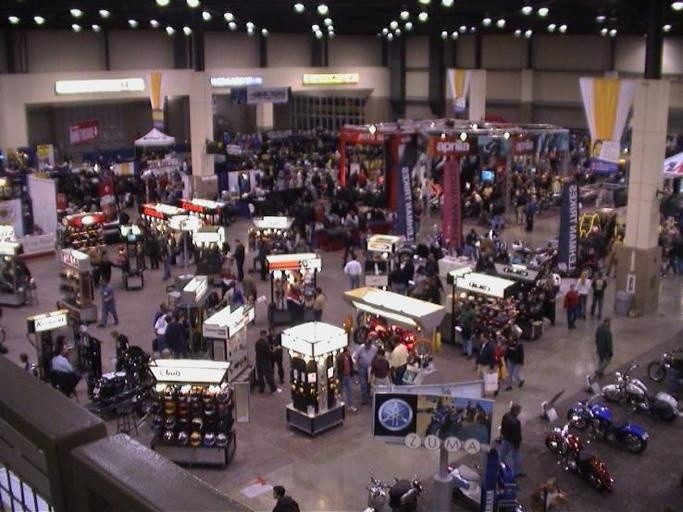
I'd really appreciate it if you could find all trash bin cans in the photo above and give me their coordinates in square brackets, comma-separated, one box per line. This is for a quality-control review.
[615, 290, 634, 317]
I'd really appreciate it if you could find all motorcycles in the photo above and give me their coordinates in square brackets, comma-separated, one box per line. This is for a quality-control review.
[646, 345, 683, 392]
[543, 415, 616, 494]
[567, 392, 650, 455]
[427, 405, 465, 438]
[600, 360, 683, 422]
[89, 340, 158, 409]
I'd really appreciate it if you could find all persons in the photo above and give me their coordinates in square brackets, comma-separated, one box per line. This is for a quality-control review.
[0, 137, 683, 411]
[499, 402, 530, 477]
[271, 485, 301, 512]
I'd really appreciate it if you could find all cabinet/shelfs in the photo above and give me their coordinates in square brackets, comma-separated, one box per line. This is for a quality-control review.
[0, 196, 544, 468]
